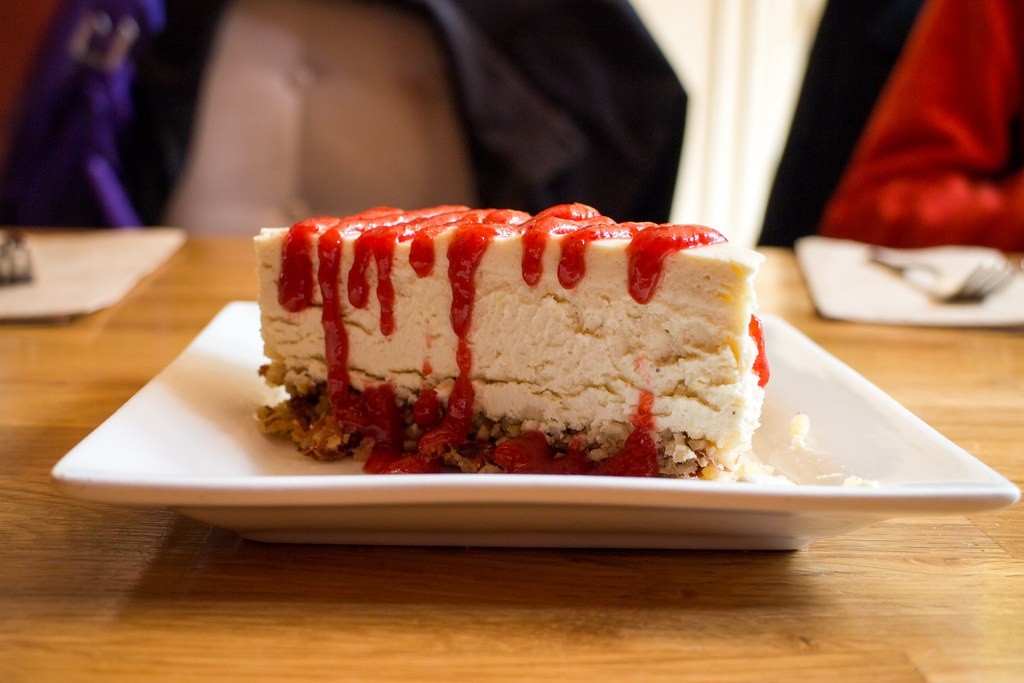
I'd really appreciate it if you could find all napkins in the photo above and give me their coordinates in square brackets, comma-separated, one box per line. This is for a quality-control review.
[0, 229, 186, 319]
[796, 233, 1024, 330]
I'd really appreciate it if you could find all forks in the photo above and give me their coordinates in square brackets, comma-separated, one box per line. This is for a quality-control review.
[870, 255, 1024, 308]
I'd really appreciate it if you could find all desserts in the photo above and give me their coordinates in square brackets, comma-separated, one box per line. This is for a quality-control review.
[252, 201, 770, 479]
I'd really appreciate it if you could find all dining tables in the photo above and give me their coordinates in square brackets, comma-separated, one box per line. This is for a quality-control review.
[0, 229, 1024, 682]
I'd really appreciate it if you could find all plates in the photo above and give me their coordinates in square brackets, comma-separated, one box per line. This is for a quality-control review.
[49, 300, 1021, 550]
